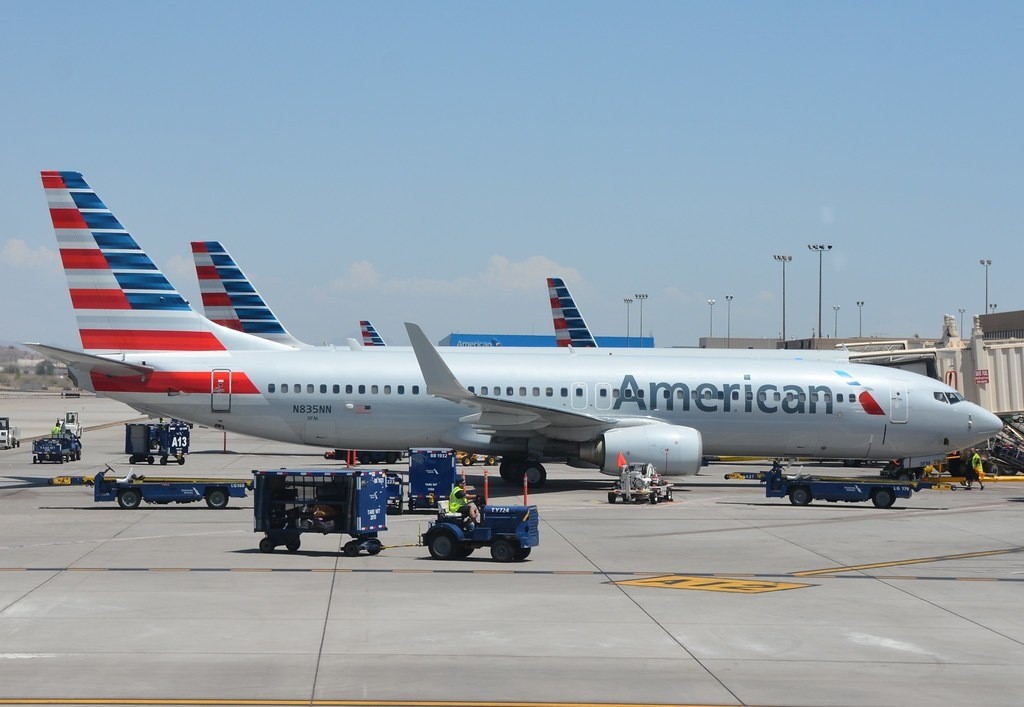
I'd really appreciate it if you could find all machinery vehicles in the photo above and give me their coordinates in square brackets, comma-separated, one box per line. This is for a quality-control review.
[252, 465, 540, 564]
[608, 462, 674, 504]
[94, 462, 253, 510]
[31, 428, 82, 465]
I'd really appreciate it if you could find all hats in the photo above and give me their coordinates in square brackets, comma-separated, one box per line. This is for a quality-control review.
[457, 478, 465, 482]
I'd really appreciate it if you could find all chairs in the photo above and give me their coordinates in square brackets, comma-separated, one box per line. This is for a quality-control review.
[437, 500, 462, 518]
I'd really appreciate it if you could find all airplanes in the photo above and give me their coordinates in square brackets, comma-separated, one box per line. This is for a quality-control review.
[19, 170, 1005, 489]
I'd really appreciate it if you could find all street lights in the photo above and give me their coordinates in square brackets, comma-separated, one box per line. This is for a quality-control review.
[708, 299, 716, 337]
[773, 254, 793, 341]
[959, 307, 966, 340]
[624, 297, 634, 348]
[635, 293, 648, 347]
[833, 304, 840, 338]
[807, 243, 833, 338]
[980, 259, 991, 315]
[725, 294, 734, 348]
[857, 301, 864, 338]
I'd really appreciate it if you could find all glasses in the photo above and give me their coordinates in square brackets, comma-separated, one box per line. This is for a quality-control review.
[461, 482, 466, 484]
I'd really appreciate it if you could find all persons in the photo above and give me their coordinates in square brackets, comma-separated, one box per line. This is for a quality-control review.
[949, 447, 984, 491]
[449, 479, 478, 526]
[52, 422, 62, 435]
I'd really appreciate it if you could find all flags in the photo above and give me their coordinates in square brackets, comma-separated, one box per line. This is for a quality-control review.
[617, 452, 626, 469]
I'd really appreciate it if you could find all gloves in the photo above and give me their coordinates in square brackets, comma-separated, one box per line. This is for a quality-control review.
[476, 494, 482, 497]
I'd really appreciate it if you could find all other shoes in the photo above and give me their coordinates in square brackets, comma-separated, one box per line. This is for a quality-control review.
[980, 485, 985, 490]
[964, 486, 972, 490]
